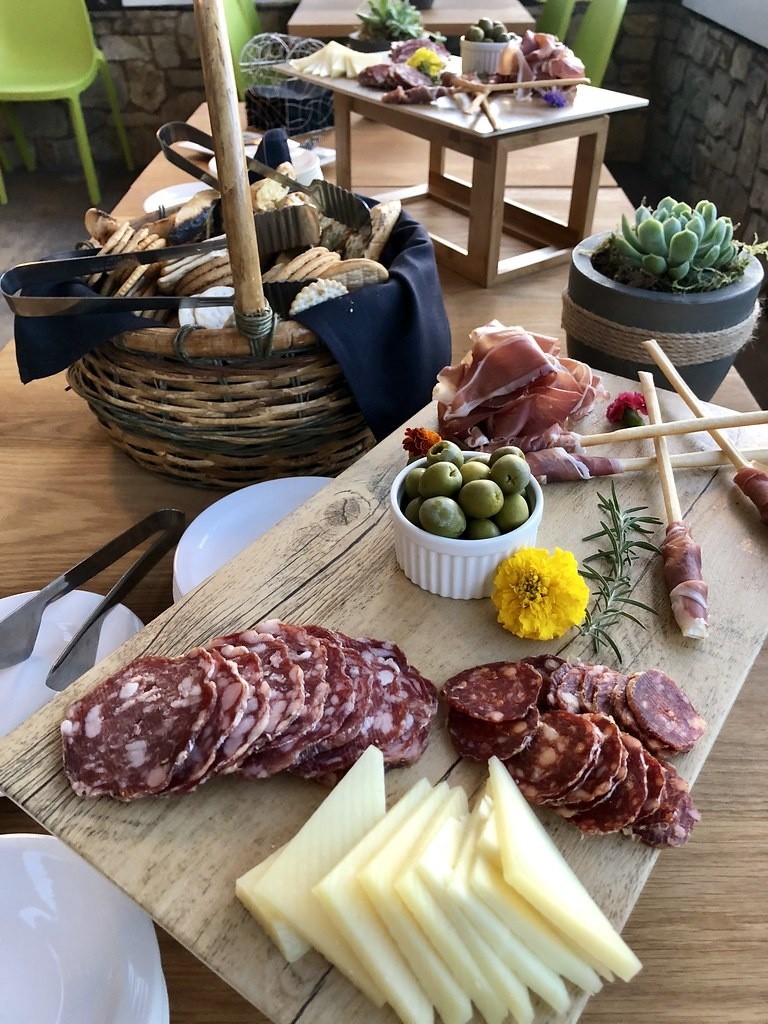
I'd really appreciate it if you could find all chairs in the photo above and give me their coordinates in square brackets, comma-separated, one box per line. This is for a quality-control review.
[223, 0, 282, 102]
[238, 0, 264, 53]
[0, 0, 134, 206]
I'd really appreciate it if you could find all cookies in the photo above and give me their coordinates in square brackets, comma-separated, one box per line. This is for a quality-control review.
[85, 220, 392, 330]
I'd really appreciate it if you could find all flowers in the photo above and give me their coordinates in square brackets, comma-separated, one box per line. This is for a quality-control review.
[402, 427, 445, 467]
[603, 392, 652, 428]
[489, 547, 592, 643]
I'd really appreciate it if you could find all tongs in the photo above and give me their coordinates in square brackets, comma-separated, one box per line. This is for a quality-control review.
[155, 121, 373, 239]
[0, 204, 320, 317]
[0, 508, 187, 692]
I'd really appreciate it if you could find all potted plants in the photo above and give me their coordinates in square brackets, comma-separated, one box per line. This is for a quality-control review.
[561, 196, 768, 402]
[458, 17, 522, 78]
[347, 0, 447, 53]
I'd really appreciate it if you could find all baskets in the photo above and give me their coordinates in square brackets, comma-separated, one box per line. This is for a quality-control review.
[67, 181, 434, 490]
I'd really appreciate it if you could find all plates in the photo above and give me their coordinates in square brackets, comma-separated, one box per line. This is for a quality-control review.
[171, 476, 335, 604]
[0, 833, 171, 1024]
[0, 589, 145, 738]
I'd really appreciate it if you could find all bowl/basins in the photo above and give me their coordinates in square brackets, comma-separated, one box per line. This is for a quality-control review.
[460, 34, 523, 73]
[388, 450, 544, 600]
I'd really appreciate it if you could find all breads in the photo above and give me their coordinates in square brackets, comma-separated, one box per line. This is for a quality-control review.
[85, 161, 402, 260]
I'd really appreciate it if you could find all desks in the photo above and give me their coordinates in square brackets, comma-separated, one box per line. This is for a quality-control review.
[0, 186, 768, 1024]
[287, 0, 537, 121]
[133, 103, 619, 186]
[271, 51, 649, 288]
